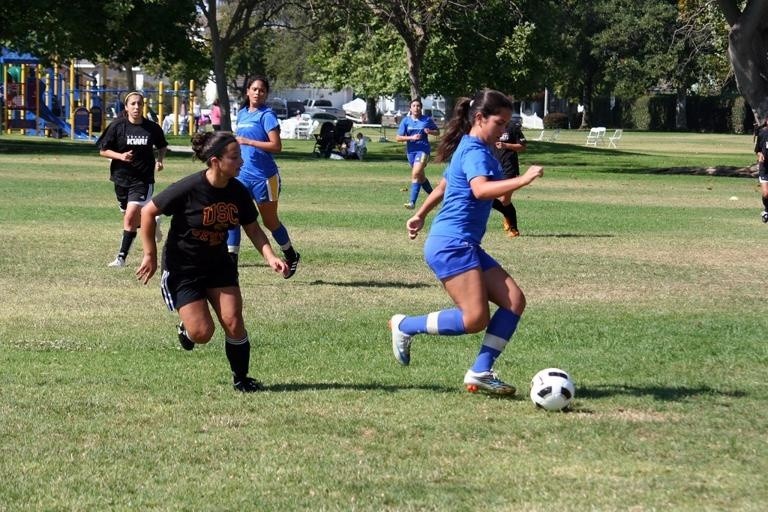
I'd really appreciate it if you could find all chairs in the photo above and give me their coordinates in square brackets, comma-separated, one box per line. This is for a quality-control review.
[597, 126, 605, 141]
[311, 121, 319, 138]
[585, 127, 599, 148]
[301, 114, 315, 122]
[608, 129, 622, 149]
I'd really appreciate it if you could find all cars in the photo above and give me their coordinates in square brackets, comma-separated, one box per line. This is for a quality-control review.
[420, 108, 445, 124]
[304, 111, 340, 134]
[306, 99, 333, 113]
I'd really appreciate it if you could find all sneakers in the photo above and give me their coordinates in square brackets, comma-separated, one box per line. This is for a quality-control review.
[502, 214, 512, 233]
[506, 227, 520, 238]
[759, 209, 767, 223]
[231, 376, 265, 393]
[107, 256, 128, 268]
[402, 202, 417, 211]
[387, 312, 414, 368]
[462, 368, 516, 398]
[154, 214, 164, 244]
[176, 321, 193, 351]
[283, 251, 300, 279]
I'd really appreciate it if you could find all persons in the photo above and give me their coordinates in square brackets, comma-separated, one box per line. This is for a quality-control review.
[193, 98, 202, 133]
[133, 132, 287, 393]
[210, 98, 223, 133]
[196, 115, 210, 133]
[227, 74, 299, 279]
[179, 98, 188, 136]
[352, 132, 367, 161]
[389, 88, 544, 399]
[491, 119, 528, 237]
[395, 99, 441, 209]
[755, 111, 768, 223]
[95, 92, 168, 269]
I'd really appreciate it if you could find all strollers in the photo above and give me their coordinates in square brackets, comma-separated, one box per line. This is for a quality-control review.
[311, 118, 353, 162]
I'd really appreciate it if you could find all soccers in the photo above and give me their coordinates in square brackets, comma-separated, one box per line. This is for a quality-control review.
[531, 368, 573, 412]
